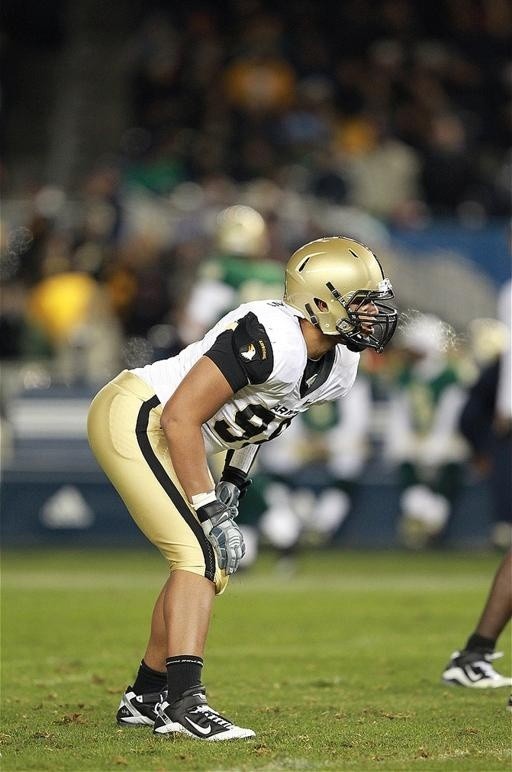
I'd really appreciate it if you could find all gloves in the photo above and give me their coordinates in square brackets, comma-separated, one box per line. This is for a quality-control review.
[191, 470, 252, 576]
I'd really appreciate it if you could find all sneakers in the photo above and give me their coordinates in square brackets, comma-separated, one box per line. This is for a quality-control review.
[116, 684, 256, 742]
[441, 651, 512, 688]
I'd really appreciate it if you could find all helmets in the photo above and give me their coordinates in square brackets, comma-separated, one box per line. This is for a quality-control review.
[282, 235, 388, 336]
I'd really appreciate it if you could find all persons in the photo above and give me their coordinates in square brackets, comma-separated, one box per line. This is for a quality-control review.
[441, 550, 512, 712]
[87, 234, 400, 743]
[2, 1, 510, 554]
